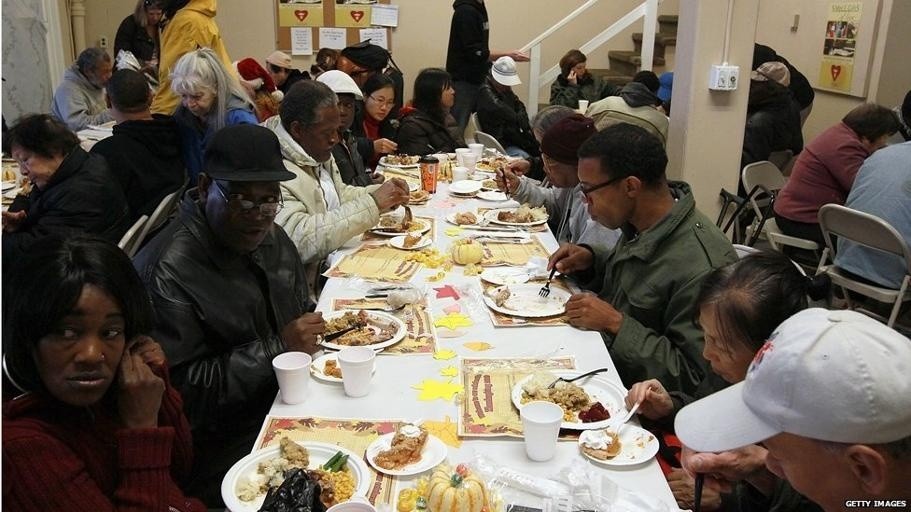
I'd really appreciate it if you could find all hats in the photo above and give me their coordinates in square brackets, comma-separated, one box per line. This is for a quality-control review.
[265, 50, 293, 69]
[654, 71, 674, 102]
[315, 69, 363, 101]
[234, 58, 285, 102]
[203, 121, 298, 183]
[491, 55, 522, 87]
[674, 306, 910, 455]
[540, 114, 598, 166]
[749, 61, 792, 86]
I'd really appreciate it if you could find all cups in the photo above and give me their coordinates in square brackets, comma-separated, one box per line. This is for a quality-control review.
[272, 351, 313, 404]
[418, 142, 486, 194]
[520, 400, 565, 461]
[327, 501, 379, 511]
[578, 100, 590, 114]
[339, 346, 377, 397]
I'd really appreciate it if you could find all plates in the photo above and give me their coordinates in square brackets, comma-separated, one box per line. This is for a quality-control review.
[316, 310, 409, 350]
[2, 182, 24, 199]
[481, 268, 531, 285]
[484, 284, 573, 318]
[310, 352, 378, 383]
[221, 442, 370, 512]
[578, 422, 661, 466]
[513, 369, 634, 430]
[371, 154, 549, 236]
[390, 236, 433, 250]
[366, 431, 450, 476]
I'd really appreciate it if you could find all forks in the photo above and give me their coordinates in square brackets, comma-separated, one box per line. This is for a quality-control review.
[616, 386, 652, 436]
[539, 267, 557, 297]
[547, 368, 609, 389]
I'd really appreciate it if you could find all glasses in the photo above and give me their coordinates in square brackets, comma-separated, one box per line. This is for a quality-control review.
[579, 176, 620, 203]
[211, 178, 285, 218]
[370, 94, 395, 108]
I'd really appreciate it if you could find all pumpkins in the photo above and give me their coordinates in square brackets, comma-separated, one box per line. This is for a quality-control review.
[452, 237, 483, 264]
[424, 464, 488, 512]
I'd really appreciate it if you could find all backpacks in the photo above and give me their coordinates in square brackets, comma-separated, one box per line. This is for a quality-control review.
[341, 38, 391, 71]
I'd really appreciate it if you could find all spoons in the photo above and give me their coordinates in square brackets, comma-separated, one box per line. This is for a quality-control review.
[366, 304, 406, 312]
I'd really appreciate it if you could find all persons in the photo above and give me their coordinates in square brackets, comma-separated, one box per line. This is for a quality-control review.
[526, 40, 911, 511]
[396, 66, 470, 153]
[445, 0, 492, 144]
[474, 54, 546, 182]
[1, 0, 403, 511]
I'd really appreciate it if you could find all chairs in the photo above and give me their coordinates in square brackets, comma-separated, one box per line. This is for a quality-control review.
[473, 130, 508, 158]
[132, 173, 189, 258]
[816, 202, 910, 332]
[731, 149, 793, 243]
[743, 159, 824, 274]
[117, 214, 147, 256]
